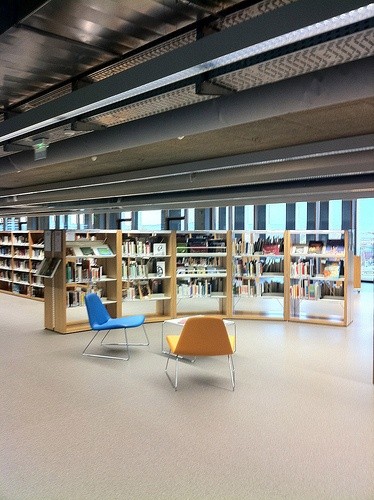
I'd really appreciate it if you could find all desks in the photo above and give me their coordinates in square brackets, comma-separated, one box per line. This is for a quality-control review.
[162, 315, 236, 363]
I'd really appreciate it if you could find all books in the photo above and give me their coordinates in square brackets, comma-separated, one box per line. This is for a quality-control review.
[0, 234, 346, 309]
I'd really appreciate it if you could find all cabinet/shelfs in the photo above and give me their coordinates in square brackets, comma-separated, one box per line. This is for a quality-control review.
[0, 230, 44, 302]
[44, 230, 353, 334]
[353, 255, 361, 292]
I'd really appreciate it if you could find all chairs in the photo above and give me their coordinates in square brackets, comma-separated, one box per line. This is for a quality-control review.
[82, 293, 150, 361]
[164, 317, 237, 391]
